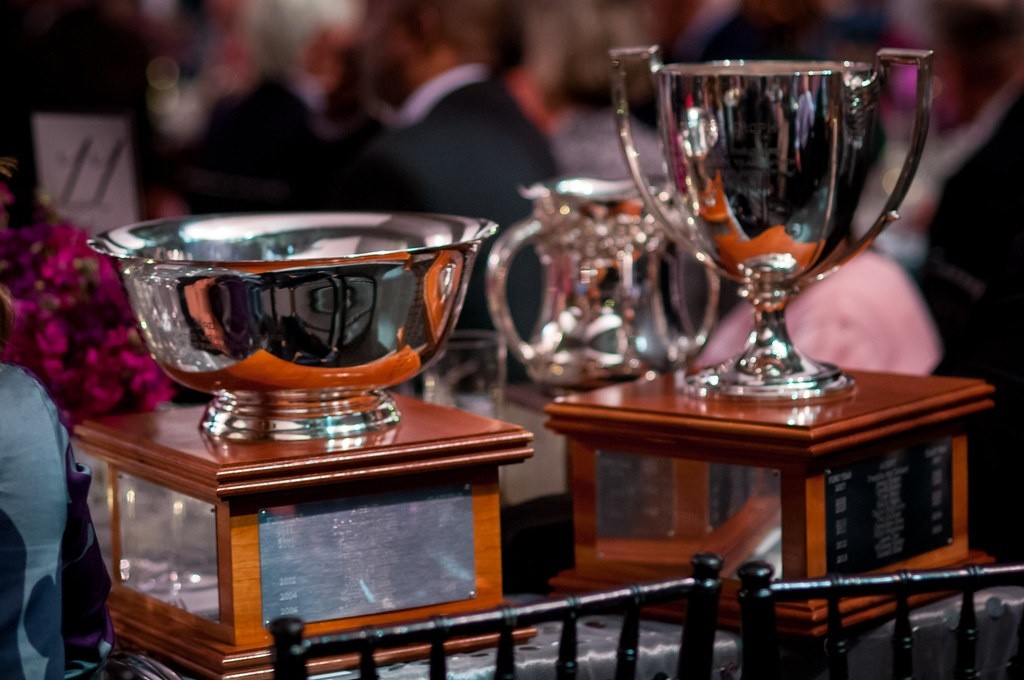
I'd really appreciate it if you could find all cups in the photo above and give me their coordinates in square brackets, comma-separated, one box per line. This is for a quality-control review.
[608, 43, 935, 407]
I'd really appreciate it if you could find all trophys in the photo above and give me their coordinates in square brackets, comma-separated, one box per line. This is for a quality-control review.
[62, 209, 537, 680]
[490, 176, 719, 420]
[546, 41, 1000, 629]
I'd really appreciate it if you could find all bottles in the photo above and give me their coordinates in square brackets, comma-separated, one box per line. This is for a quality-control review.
[484, 174, 719, 407]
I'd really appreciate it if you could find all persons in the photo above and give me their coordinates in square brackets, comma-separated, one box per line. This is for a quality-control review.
[120, 0, 1024, 581]
[0, 109, 168, 680]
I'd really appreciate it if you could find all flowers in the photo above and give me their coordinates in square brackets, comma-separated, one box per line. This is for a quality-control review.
[0, 158, 179, 420]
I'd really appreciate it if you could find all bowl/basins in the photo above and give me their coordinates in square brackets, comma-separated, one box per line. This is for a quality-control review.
[83, 209, 499, 441]
